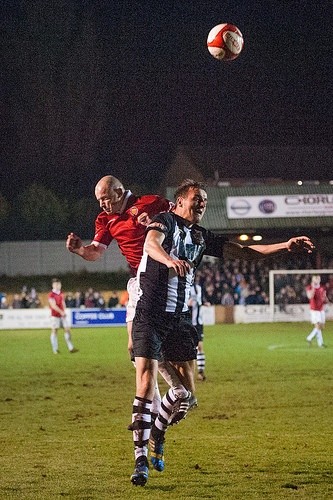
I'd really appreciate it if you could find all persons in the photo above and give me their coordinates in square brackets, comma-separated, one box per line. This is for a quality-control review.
[125, 179, 315, 488]
[63, 173, 197, 432]
[187, 279, 206, 380]
[0, 242, 333, 354]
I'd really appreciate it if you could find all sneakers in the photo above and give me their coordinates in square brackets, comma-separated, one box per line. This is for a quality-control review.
[130, 461, 149, 486]
[167, 395, 198, 426]
[128, 412, 159, 430]
[147, 434, 166, 472]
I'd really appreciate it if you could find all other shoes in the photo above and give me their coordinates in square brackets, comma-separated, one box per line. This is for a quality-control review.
[304, 338, 312, 346]
[318, 343, 327, 349]
[69, 348, 79, 353]
[197, 369, 205, 380]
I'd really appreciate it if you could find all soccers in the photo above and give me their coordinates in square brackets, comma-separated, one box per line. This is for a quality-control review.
[207, 24, 244, 62]
